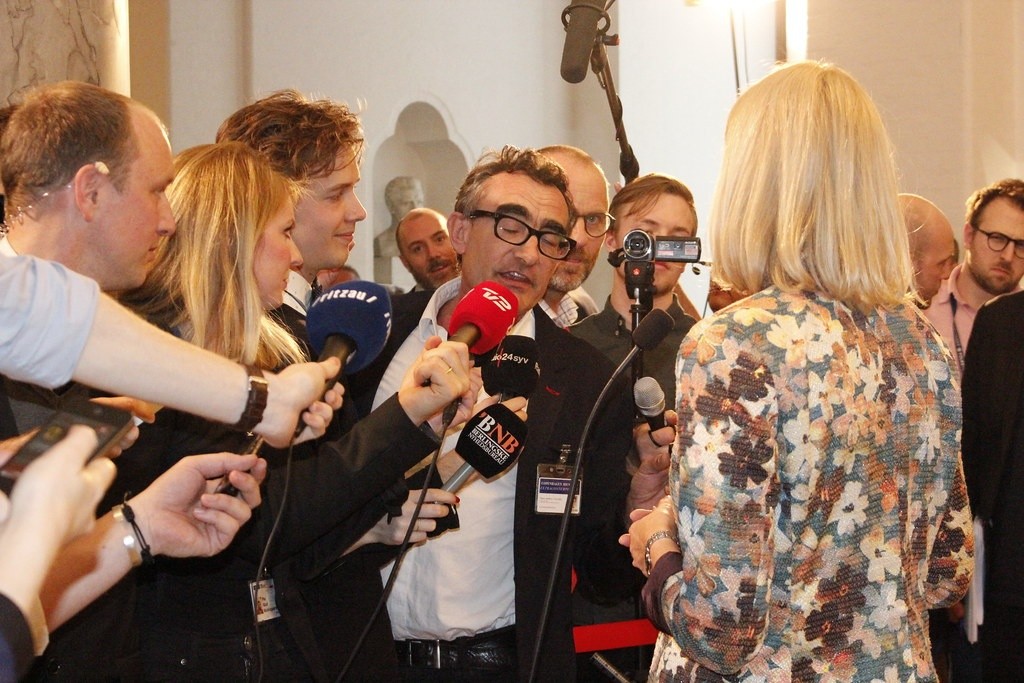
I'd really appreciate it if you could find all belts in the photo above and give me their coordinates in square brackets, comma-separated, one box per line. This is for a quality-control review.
[391, 639, 520, 671]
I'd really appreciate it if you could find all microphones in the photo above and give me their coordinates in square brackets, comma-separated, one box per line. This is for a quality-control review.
[292, 280, 391, 437]
[560, 0, 606, 84]
[634, 376, 666, 432]
[418, 280, 539, 508]
[528, 308, 675, 683]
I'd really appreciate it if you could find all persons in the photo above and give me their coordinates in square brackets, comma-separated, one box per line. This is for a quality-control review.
[620, 61, 974, 683]
[0, 83, 697, 683]
[896, 180, 1024, 683]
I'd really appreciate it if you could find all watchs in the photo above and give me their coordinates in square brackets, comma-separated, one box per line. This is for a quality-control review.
[644, 531, 680, 574]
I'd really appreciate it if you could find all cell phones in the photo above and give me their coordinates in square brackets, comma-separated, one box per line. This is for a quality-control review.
[0, 395, 134, 496]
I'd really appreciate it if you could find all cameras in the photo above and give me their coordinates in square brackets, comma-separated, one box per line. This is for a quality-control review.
[622, 229, 702, 264]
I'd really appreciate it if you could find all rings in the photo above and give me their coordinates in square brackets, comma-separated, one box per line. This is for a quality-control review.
[417, 519, 420, 530]
[446, 368, 452, 373]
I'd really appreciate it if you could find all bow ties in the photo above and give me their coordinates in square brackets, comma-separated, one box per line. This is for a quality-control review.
[308, 276, 324, 303]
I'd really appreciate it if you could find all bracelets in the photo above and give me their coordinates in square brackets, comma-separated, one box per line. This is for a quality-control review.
[230, 363, 269, 434]
[122, 498, 160, 579]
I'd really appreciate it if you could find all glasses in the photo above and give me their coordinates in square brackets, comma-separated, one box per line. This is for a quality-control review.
[569, 212, 615, 238]
[973, 227, 1024, 259]
[469, 209, 577, 260]
